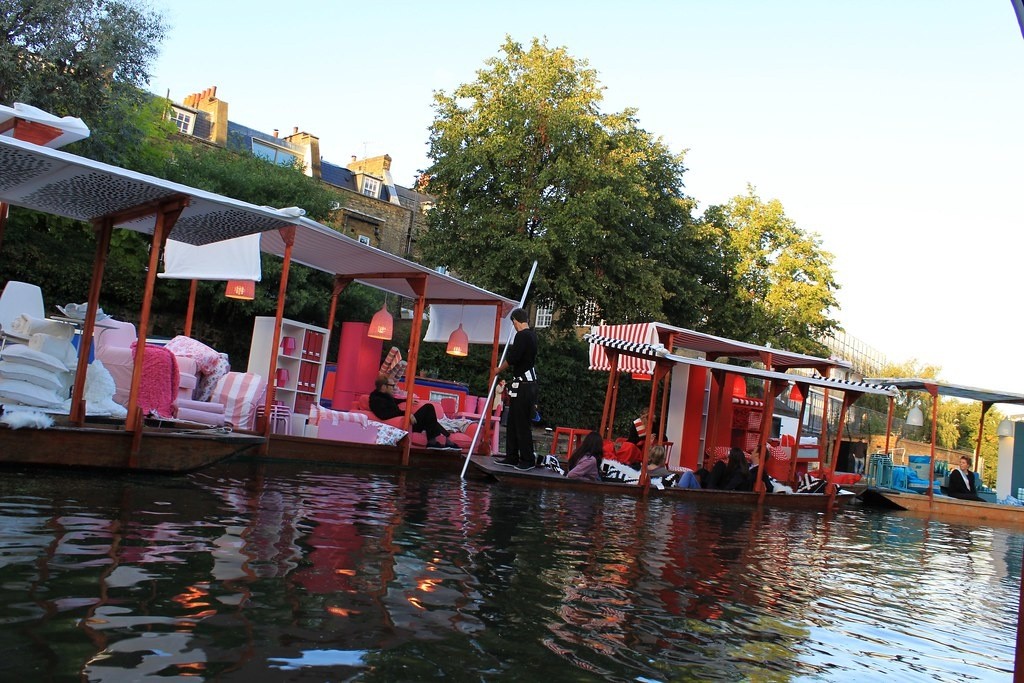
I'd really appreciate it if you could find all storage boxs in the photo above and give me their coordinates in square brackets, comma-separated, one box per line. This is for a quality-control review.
[732, 430, 760, 452]
[733, 410, 762, 432]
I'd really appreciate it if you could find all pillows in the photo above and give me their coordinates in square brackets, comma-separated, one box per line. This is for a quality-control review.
[308, 404, 409, 445]
[0, 342, 69, 408]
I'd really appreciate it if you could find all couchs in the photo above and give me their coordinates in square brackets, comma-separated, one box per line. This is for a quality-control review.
[350, 394, 485, 454]
[93, 318, 197, 407]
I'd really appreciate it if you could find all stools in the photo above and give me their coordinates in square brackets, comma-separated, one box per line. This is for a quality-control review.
[550, 427, 592, 459]
[867, 453, 907, 490]
[252, 404, 292, 436]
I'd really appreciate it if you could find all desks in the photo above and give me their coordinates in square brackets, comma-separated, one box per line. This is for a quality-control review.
[51, 315, 121, 363]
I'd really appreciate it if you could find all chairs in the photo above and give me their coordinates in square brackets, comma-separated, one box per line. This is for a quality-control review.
[0, 280, 46, 353]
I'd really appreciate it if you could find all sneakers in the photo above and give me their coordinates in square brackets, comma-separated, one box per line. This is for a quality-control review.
[513, 459, 535, 471]
[445, 443, 462, 451]
[426, 442, 450, 450]
[494, 457, 519, 466]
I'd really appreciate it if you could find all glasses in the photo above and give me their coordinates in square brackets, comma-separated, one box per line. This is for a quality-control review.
[386, 384, 395, 388]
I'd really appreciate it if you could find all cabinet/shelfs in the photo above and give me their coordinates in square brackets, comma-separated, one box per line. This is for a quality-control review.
[247, 316, 330, 413]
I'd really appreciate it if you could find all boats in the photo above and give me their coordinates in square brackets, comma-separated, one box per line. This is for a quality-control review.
[0, 100, 1023, 532]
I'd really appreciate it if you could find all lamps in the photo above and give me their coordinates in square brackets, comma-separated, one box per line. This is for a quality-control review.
[224, 279, 254, 300]
[367, 292, 393, 339]
[447, 304, 468, 356]
[996, 415, 1013, 437]
[905, 392, 923, 426]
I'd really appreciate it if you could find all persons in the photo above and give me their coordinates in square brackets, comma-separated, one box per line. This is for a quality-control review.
[948, 455, 987, 502]
[693, 444, 773, 493]
[493, 379, 507, 428]
[627, 411, 669, 451]
[566, 434, 606, 482]
[640, 447, 702, 490]
[493, 308, 538, 471]
[852, 437, 868, 477]
[368, 375, 459, 448]
